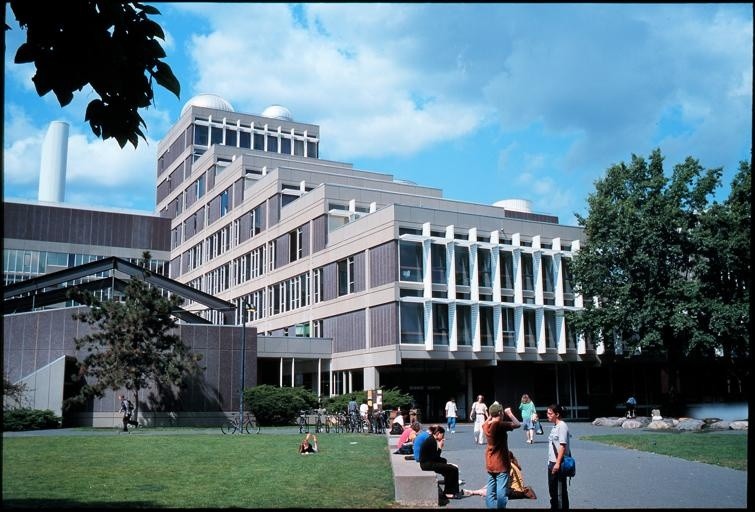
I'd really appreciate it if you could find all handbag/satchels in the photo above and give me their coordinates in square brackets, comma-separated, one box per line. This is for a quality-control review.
[524, 487, 536, 498]
[561, 455, 575, 477]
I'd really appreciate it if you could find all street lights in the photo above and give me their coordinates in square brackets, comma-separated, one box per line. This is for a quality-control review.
[238, 298, 256, 435]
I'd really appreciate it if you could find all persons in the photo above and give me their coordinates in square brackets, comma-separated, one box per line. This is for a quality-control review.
[299, 433, 318, 454]
[625, 396, 637, 418]
[519, 395, 536, 444]
[118, 394, 138, 432]
[470, 395, 489, 444]
[482, 402, 521, 508]
[546, 404, 572, 510]
[463, 450, 524, 498]
[348, 395, 462, 499]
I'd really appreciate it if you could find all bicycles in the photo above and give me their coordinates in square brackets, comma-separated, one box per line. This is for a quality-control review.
[295, 407, 386, 435]
[220, 412, 261, 436]
